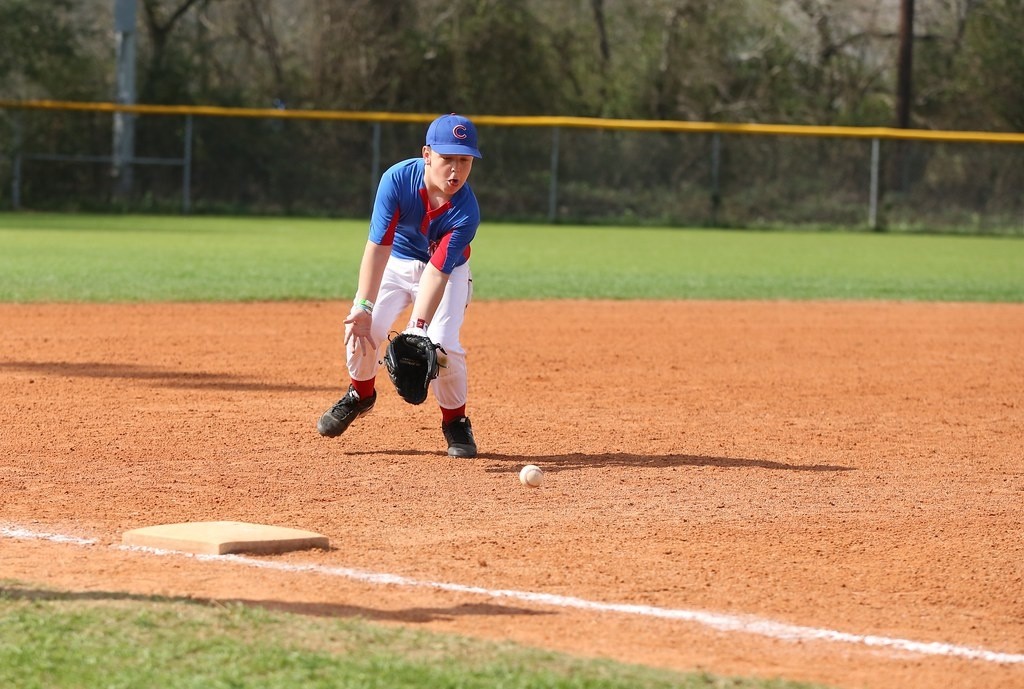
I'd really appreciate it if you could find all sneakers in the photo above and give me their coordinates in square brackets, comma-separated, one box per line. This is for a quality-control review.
[441, 416, 478, 458]
[317, 383, 376, 438]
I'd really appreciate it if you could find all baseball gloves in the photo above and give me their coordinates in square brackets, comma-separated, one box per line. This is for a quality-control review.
[384, 331, 447, 406]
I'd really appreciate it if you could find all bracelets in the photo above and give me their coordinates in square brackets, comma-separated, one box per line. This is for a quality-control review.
[351, 304, 372, 315]
[407, 318, 429, 334]
[353, 298, 374, 311]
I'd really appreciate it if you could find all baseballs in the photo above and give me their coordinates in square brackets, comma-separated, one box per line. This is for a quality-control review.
[519, 464, 545, 489]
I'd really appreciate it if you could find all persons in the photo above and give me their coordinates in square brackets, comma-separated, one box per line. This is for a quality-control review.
[319, 112, 482, 458]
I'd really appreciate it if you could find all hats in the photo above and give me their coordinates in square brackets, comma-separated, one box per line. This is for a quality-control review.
[426, 113, 483, 159]
